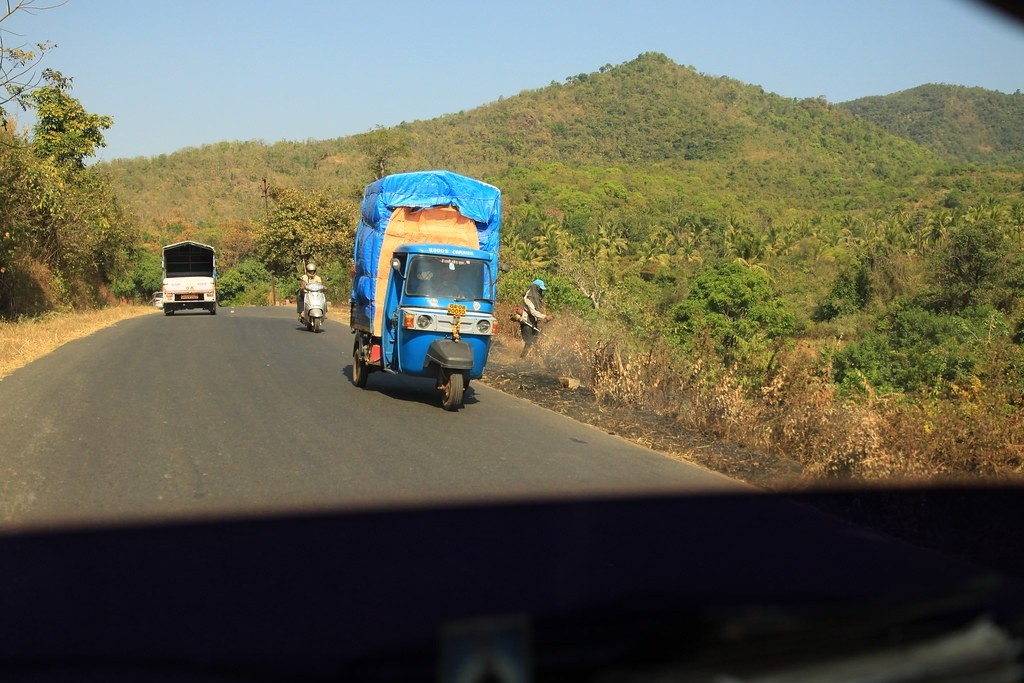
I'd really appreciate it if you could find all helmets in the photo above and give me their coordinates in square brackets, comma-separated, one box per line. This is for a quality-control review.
[306, 263, 317, 277]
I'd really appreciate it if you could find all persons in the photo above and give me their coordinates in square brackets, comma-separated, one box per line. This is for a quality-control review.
[520, 279, 553, 359]
[297, 263, 328, 320]
[415, 268, 465, 299]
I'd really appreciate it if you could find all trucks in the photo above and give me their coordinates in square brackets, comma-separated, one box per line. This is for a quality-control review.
[162, 241, 217, 317]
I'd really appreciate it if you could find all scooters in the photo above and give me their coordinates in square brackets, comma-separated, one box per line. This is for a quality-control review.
[294, 283, 326, 333]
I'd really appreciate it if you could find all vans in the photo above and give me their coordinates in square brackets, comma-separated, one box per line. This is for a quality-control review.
[152, 291, 164, 308]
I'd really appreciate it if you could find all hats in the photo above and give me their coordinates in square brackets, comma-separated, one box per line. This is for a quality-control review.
[532, 279, 546, 290]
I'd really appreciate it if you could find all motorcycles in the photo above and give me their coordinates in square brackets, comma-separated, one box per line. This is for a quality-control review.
[349, 244, 509, 411]
